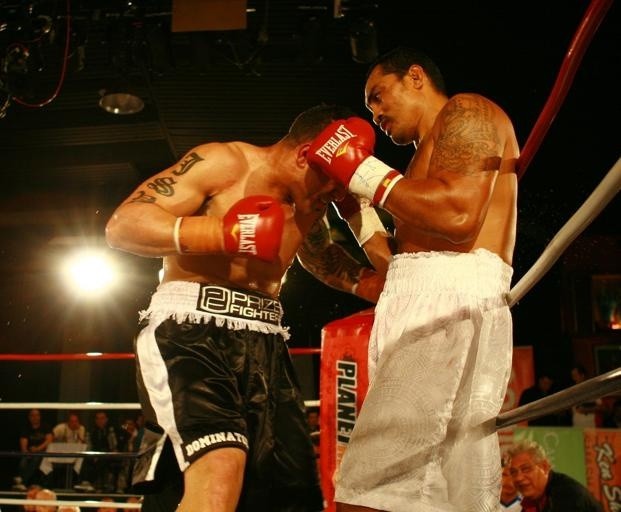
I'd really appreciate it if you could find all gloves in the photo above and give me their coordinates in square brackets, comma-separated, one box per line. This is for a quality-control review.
[307, 114, 404, 247]
[174, 195, 283, 265]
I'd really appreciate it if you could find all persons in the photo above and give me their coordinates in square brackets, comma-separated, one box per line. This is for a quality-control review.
[104, 103, 395, 511]
[304, 45, 522, 509]
